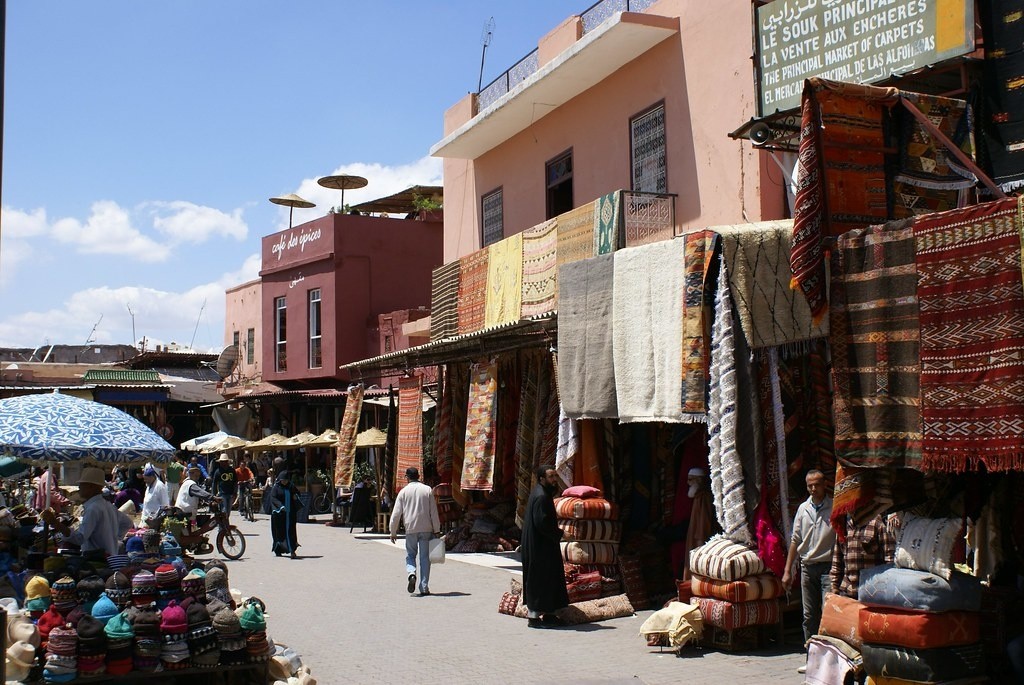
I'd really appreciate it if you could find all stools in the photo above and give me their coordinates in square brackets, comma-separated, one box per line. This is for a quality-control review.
[378, 513, 391, 534]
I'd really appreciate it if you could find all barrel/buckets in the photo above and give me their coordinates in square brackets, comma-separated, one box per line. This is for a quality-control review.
[429, 534, 445, 564]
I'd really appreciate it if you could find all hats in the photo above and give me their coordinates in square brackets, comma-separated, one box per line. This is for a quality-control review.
[216, 454, 233, 463]
[76, 468, 105, 486]
[0, 529, 275, 685]
[188, 467, 200, 473]
[144, 469, 154, 476]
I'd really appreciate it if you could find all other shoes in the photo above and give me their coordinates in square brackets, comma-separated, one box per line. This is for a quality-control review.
[528, 617, 543, 627]
[276, 552, 282, 556]
[291, 553, 296, 559]
[408, 575, 416, 593]
[797, 664, 807, 672]
[241, 512, 244, 516]
[420, 591, 430, 596]
[541, 615, 567, 628]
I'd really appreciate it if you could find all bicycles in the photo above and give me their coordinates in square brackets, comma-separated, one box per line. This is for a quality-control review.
[314, 475, 333, 513]
[238, 479, 255, 522]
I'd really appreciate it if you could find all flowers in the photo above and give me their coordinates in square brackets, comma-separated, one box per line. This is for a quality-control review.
[311, 469, 326, 483]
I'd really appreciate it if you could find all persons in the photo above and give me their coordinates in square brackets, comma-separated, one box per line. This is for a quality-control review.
[110, 452, 282, 531]
[0, 462, 73, 524]
[782, 469, 835, 674]
[270, 471, 302, 558]
[683, 469, 719, 583]
[389, 468, 441, 596]
[352, 474, 398, 532]
[41, 468, 132, 565]
[521, 467, 568, 629]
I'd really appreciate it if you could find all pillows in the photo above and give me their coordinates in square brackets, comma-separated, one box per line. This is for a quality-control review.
[893, 512, 964, 580]
[561, 485, 601, 498]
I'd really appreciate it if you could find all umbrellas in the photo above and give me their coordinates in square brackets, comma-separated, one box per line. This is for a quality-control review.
[269, 194, 316, 228]
[0, 393, 177, 511]
[182, 431, 243, 452]
[200, 429, 400, 521]
[317, 173, 368, 215]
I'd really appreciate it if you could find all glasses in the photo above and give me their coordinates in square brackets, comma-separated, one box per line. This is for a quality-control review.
[405, 474, 408, 478]
[366, 478, 371, 481]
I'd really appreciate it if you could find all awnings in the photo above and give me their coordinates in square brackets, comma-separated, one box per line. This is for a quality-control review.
[340, 306, 560, 403]
[346, 183, 444, 214]
[200, 387, 439, 414]
[0, 384, 176, 392]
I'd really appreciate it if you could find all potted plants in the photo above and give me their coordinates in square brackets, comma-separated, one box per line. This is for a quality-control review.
[410, 186, 443, 222]
[337, 203, 350, 215]
[162, 515, 184, 545]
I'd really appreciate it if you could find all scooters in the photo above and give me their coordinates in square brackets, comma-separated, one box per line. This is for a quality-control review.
[180, 498, 245, 559]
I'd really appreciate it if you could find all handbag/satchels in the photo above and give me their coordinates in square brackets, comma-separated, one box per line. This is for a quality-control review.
[428, 538, 445, 564]
[293, 493, 305, 511]
